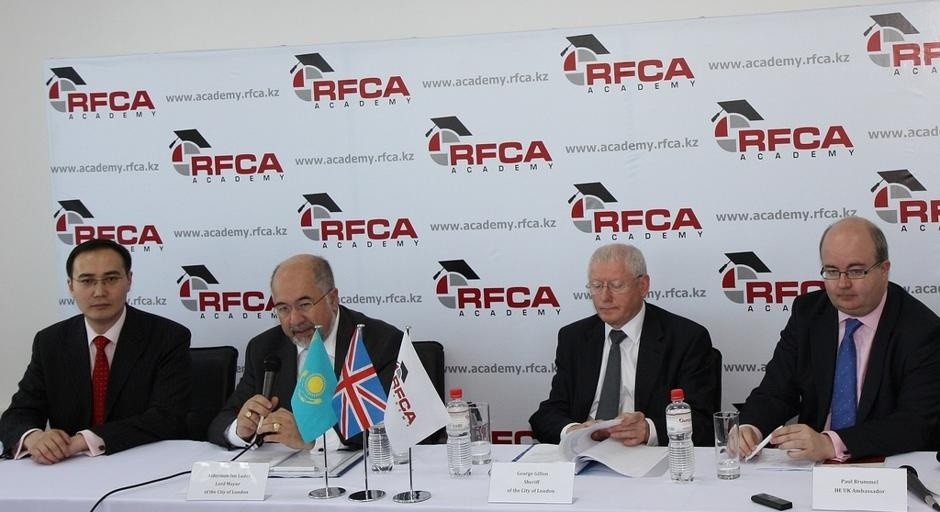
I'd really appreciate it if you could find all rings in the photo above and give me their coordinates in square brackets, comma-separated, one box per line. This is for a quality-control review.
[246, 411, 252, 417]
[273, 422, 280, 432]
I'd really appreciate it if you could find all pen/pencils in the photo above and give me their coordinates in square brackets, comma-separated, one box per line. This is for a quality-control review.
[745, 423, 783, 465]
[268, 465, 320, 473]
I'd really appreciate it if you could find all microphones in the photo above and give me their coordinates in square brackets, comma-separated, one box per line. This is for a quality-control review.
[896, 464, 936, 512]
[255, 352, 280, 435]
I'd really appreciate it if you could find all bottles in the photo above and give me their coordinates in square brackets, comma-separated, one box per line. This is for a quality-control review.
[444, 388, 473, 480]
[368, 421, 395, 473]
[665, 388, 696, 484]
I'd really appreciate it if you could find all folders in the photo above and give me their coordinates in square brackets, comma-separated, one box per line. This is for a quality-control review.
[205, 442, 369, 479]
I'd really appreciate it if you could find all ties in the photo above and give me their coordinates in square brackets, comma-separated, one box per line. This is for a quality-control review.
[596, 330, 628, 420]
[829, 320, 863, 429]
[92, 336, 111, 426]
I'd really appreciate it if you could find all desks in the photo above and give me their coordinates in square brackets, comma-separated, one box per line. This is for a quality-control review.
[0, 444, 940, 512]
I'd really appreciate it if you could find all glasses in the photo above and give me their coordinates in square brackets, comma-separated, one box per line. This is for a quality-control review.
[821, 256, 887, 280]
[272, 287, 331, 314]
[586, 274, 642, 295]
[70, 270, 129, 285]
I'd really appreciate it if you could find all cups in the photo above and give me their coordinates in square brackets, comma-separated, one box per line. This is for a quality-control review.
[468, 402, 492, 464]
[715, 412, 741, 480]
[391, 447, 410, 465]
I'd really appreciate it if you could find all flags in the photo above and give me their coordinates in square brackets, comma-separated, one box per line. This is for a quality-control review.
[291, 325, 338, 443]
[331, 328, 387, 438]
[383, 325, 451, 456]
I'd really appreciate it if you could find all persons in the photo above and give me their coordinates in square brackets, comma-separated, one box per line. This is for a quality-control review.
[726, 217, 940, 462]
[528, 243, 713, 446]
[207, 255, 404, 456]
[0, 239, 192, 466]
